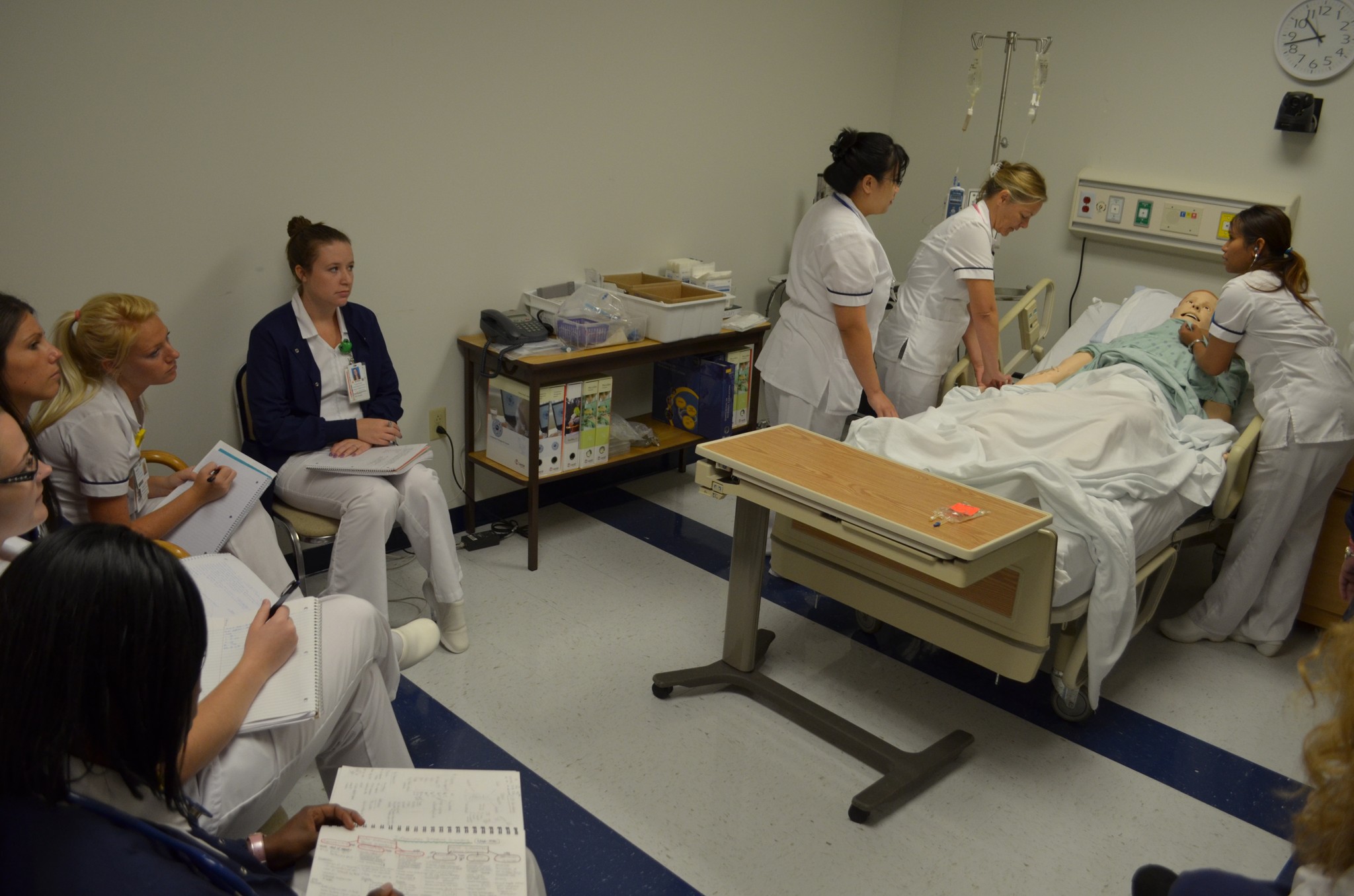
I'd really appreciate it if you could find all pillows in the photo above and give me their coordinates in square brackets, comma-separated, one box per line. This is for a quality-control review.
[1090, 286, 1255, 389]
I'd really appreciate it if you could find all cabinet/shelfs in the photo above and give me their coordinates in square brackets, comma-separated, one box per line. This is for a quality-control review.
[456, 322, 760, 571]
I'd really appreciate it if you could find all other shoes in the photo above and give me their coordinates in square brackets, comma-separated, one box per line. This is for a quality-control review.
[390, 617, 442, 670]
[423, 576, 468, 654]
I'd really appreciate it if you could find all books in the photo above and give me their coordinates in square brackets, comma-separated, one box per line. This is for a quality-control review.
[303, 766, 526, 896]
[176, 554, 283, 621]
[196, 597, 324, 734]
[304, 443, 434, 476]
[152, 440, 276, 555]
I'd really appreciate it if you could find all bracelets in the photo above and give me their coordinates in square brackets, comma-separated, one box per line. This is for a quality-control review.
[1345, 547, 1354, 557]
[1188, 339, 1204, 353]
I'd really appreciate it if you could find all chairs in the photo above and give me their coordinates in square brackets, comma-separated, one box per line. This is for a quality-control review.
[233, 362, 400, 599]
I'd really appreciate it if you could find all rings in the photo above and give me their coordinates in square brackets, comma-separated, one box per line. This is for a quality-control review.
[353, 445, 358, 449]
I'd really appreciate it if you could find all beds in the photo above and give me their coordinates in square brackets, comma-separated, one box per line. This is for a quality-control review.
[770, 279, 1263, 723]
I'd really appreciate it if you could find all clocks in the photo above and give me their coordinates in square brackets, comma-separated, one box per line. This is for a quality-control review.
[1274, 0, 1354, 82]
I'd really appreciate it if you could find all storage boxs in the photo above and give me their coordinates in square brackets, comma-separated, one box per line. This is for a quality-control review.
[524, 266, 743, 352]
[608, 436, 631, 459]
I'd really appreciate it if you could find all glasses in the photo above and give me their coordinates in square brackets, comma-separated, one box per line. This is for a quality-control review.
[0, 447, 38, 484]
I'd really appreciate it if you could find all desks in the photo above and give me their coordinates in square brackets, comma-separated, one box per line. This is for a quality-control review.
[652, 423, 1053, 828]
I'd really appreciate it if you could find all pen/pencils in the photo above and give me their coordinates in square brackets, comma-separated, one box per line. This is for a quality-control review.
[207, 468, 222, 483]
[266, 577, 303, 620]
[389, 420, 398, 446]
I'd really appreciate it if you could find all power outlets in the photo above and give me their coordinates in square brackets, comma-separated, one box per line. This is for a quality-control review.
[429, 407, 447, 442]
[1077, 190, 1096, 219]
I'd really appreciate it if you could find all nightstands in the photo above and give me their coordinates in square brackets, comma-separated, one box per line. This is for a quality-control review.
[1294, 455, 1354, 627]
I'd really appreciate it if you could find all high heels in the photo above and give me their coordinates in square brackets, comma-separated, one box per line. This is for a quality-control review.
[1159, 612, 1228, 643]
[1232, 630, 1283, 657]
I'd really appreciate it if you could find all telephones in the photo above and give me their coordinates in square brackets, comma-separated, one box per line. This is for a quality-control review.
[480, 307, 548, 345]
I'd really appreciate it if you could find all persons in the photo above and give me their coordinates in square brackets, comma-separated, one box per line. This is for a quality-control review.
[353, 368, 361, 380]
[1157, 204, 1354, 658]
[246, 215, 470, 654]
[142, 462, 147, 475]
[1283, 500, 1354, 896]
[0, 292, 543, 896]
[873, 161, 1048, 420]
[755, 128, 909, 578]
[1014, 290, 1249, 462]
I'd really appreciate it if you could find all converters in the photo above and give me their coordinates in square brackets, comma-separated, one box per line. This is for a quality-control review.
[460, 530, 500, 551]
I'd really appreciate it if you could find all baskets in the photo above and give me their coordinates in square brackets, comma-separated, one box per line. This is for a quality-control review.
[558, 318, 609, 348]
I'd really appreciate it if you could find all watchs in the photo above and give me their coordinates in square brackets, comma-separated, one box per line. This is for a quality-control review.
[247, 832, 268, 866]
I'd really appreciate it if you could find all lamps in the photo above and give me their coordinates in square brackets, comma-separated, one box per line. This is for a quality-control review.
[1274, 91, 1324, 134]
